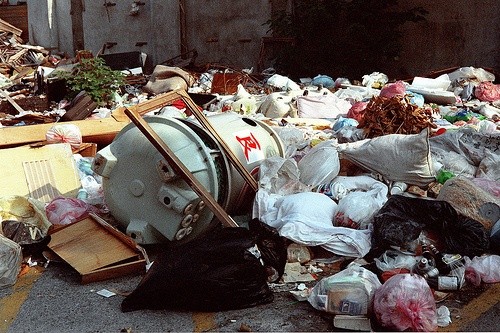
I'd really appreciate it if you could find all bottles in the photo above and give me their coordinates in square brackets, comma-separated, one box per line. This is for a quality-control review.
[410, 239, 466, 290]
